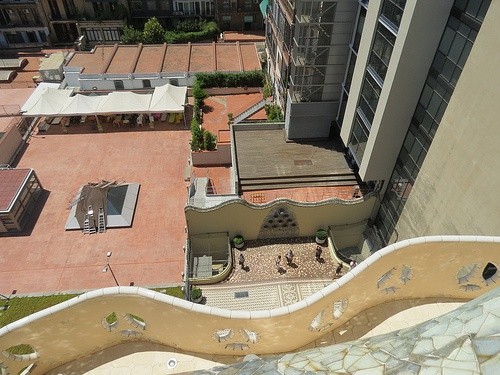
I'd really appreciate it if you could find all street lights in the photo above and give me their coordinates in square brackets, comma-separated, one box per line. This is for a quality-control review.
[101, 251, 119, 286]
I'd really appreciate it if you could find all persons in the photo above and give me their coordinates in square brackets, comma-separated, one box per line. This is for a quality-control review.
[315, 245, 322, 261]
[275, 255, 281, 267]
[333, 259, 359, 278]
[286, 250, 294, 264]
[238, 253, 245, 268]
